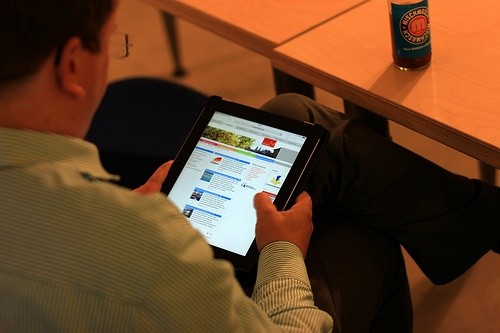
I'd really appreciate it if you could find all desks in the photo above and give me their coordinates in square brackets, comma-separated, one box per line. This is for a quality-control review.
[147, 0, 368, 96]
[273, 0, 500, 181]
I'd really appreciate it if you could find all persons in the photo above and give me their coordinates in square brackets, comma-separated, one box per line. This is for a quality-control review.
[0, 0, 500, 333]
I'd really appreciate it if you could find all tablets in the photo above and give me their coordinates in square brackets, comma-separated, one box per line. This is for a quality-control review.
[160, 96, 322, 272]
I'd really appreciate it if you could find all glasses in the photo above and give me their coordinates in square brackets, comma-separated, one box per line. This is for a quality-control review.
[54, 30, 128, 64]
